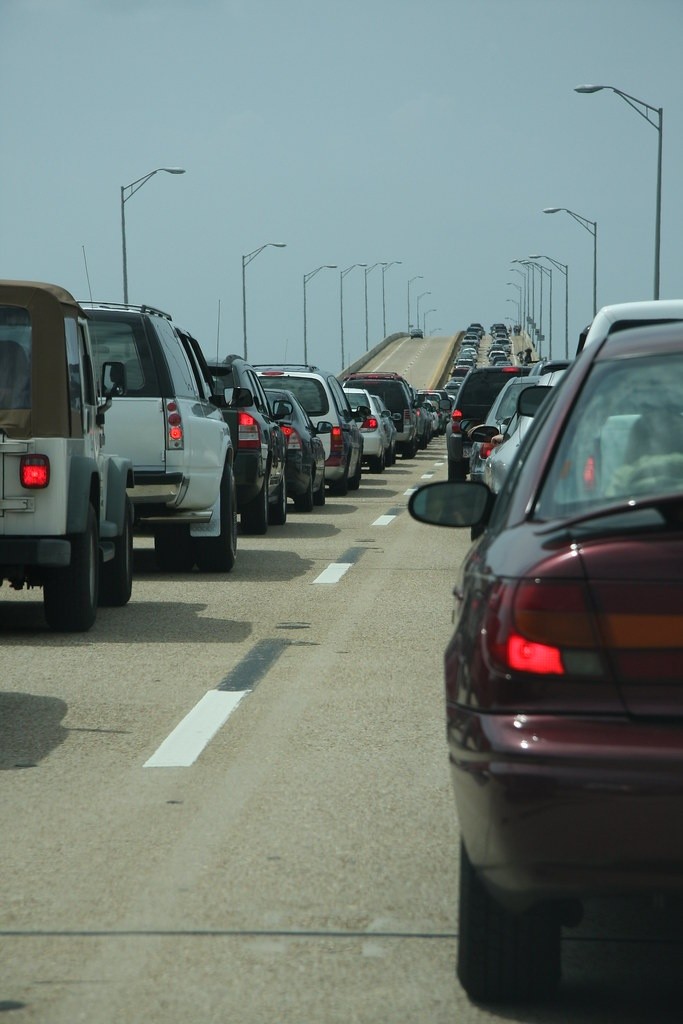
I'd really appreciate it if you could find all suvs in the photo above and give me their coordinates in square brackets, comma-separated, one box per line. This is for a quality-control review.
[207, 354, 295, 535]
[77, 298, 254, 572]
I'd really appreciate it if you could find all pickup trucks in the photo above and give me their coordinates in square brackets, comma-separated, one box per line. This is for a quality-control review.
[0, 277, 138, 631]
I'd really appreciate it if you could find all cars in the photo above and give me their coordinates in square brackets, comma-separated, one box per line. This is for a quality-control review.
[263, 387, 336, 514]
[261, 298, 683, 548]
[407, 317, 683, 924]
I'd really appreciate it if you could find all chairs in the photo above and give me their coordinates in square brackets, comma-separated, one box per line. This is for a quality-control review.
[0, 340, 32, 410]
[124, 359, 144, 389]
[624, 407, 683, 465]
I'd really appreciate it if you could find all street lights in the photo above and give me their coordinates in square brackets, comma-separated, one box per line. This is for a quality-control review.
[406, 275, 425, 332]
[416, 290, 431, 329]
[504, 259, 535, 343]
[120, 167, 186, 310]
[363, 262, 388, 353]
[574, 83, 664, 300]
[241, 242, 288, 361]
[381, 261, 403, 338]
[423, 309, 438, 336]
[302, 265, 339, 365]
[520, 260, 553, 360]
[340, 262, 367, 369]
[542, 207, 598, 319]
[527, 254, 571, 360]
